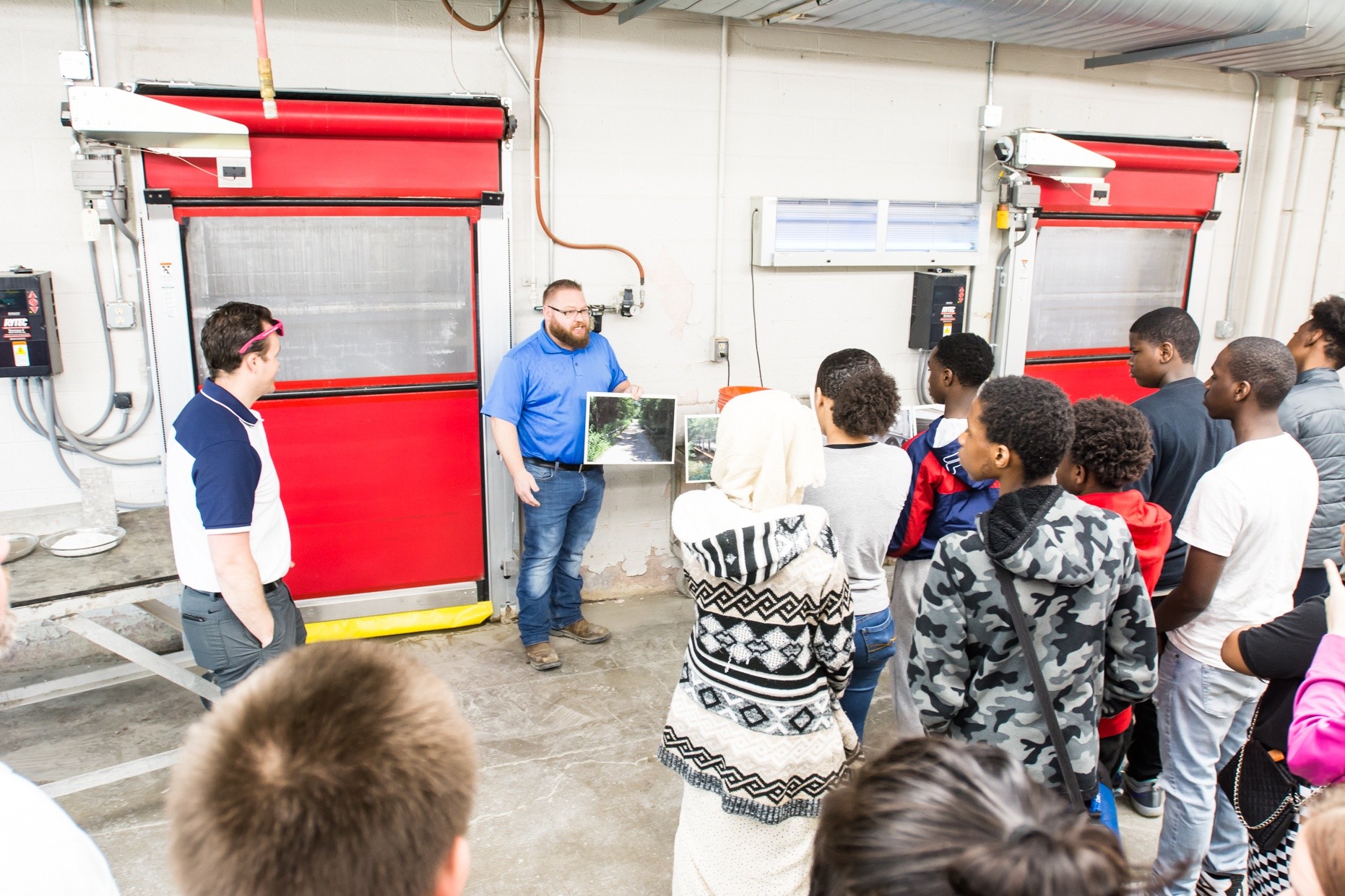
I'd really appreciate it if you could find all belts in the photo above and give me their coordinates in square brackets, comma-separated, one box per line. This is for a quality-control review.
[522, 457, 597, 473]
[189, 578, 282, 598]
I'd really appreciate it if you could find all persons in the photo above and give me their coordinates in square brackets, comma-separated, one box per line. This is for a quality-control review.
[169, 302, 307, 709]
[658, 294, 1345, 896]
[480, 280, 644, 670]
[0, 565, 122, 895]
[166, 641, 475, 896]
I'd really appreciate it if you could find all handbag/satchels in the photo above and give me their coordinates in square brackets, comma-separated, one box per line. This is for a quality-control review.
[1091, 783, 1119, 835]
[1219, 737, 1297, 851]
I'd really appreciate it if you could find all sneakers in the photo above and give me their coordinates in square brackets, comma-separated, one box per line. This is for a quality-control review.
[551, 619, 611, 643]
[526, 642, 561, 670]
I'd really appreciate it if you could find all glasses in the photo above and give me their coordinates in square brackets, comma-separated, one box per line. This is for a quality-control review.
[548, 306, 592, 319]
[238, 319, 284, 354]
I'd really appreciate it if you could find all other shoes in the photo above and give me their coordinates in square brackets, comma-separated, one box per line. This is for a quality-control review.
[1123, 763, 1163, 816]
[1196, 870, 1248, 896]
